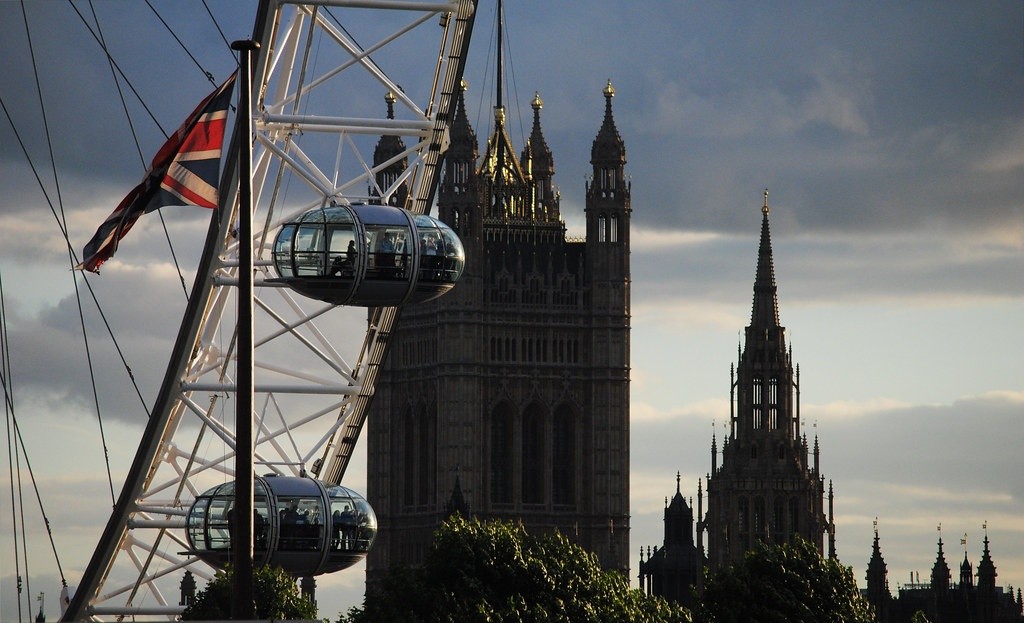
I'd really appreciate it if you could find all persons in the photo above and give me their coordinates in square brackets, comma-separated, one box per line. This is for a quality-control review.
[220, 484, 375, 565]
[314, 216, 462, 288]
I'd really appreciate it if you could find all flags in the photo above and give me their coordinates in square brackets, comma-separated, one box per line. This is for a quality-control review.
[66, 67, 243, 280]
